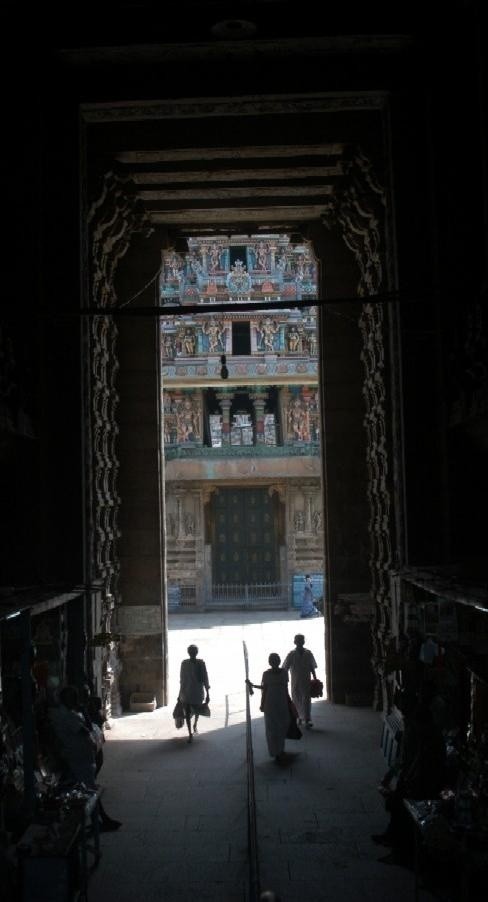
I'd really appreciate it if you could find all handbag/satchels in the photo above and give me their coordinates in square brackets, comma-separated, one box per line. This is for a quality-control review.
[173, 699, 184, 729]
[310, 680, 323, 698]
[285, 704, 303, 740]
[199, 700, 210, 717]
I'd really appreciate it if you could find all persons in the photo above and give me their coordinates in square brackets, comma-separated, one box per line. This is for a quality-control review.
[178, 645, 210, 744]
[251, 653, 292, 765]
[302, 575, 319, 616]
[281, 635, 317, 727]
[53, 686, 122, 832]
